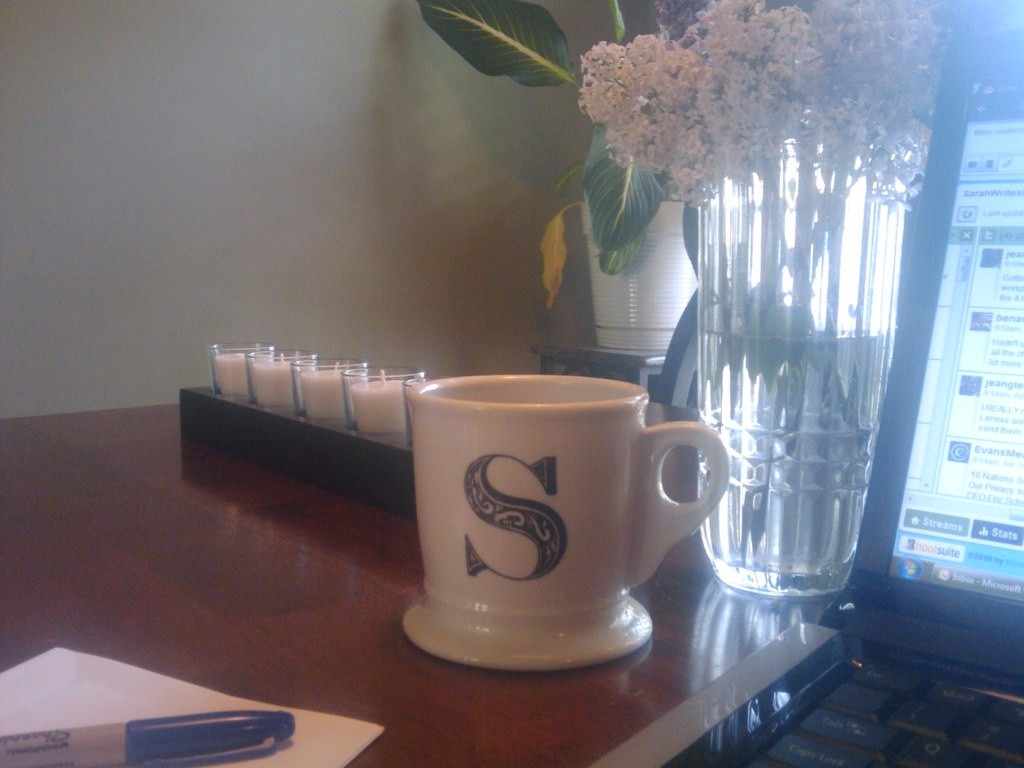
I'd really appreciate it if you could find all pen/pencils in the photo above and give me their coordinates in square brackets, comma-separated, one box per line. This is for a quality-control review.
[0, 711, 295, 768]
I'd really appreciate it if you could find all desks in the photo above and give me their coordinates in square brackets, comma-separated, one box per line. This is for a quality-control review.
[0, 403, 860, 767]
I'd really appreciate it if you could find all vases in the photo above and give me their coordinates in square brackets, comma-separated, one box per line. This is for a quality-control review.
[694, 109, 918, 600]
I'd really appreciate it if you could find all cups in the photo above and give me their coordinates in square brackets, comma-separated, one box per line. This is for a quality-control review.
[402, 374, 730, 672]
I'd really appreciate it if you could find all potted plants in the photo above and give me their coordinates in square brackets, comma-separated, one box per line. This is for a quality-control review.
[418, 1, 703, 357]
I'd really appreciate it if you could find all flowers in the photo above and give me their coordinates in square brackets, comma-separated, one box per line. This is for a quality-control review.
[572, 0, 943, 573]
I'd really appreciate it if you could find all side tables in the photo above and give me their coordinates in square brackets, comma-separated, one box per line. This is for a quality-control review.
[530, 335, 667, 400]
